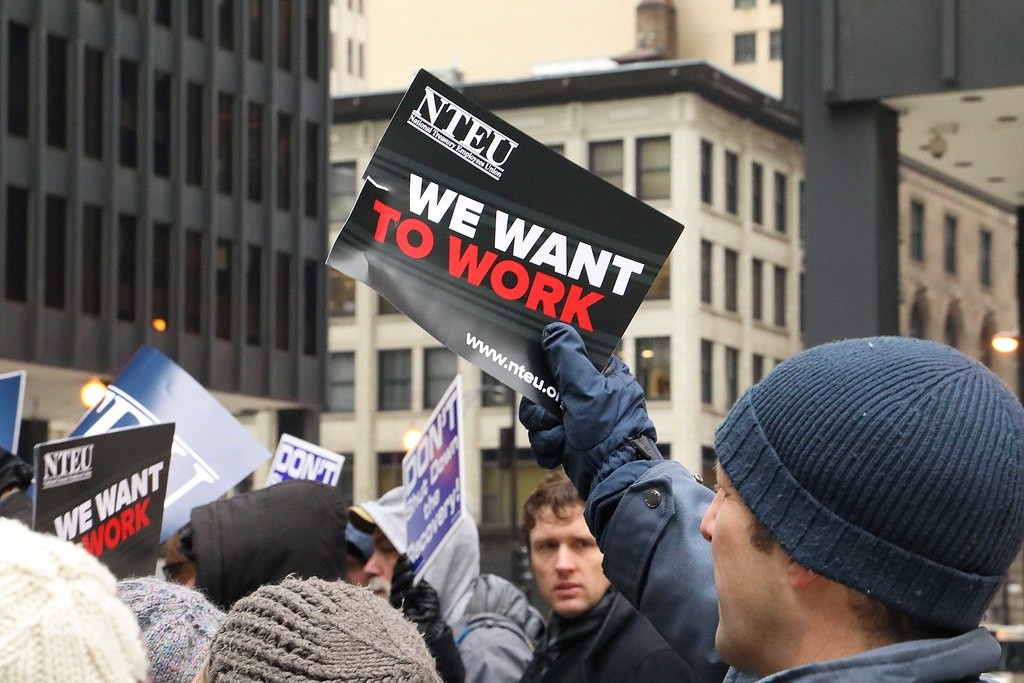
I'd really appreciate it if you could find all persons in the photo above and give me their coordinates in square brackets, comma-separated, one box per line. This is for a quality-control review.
[518, 324, 1023, 683]
[0, 394, 692, 679]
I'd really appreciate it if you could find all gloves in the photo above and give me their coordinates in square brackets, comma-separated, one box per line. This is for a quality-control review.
[518, 321, 657, 496]
[0, 445, 33, 493]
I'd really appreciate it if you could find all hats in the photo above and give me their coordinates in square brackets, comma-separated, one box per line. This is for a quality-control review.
[0, 516, 151, 683]
[208, 571, 447, 683]
[344, 521, 374, 560]
[715, 335, 1024, 627]
[118, 576, 227, 683]
